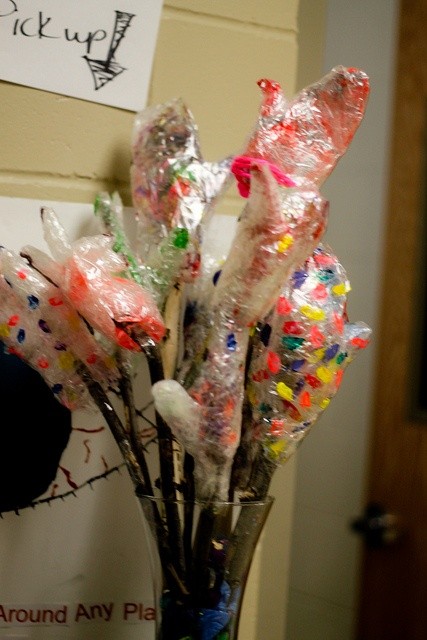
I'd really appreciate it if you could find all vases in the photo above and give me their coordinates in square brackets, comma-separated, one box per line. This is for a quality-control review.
[133, 493, 276, 639]
